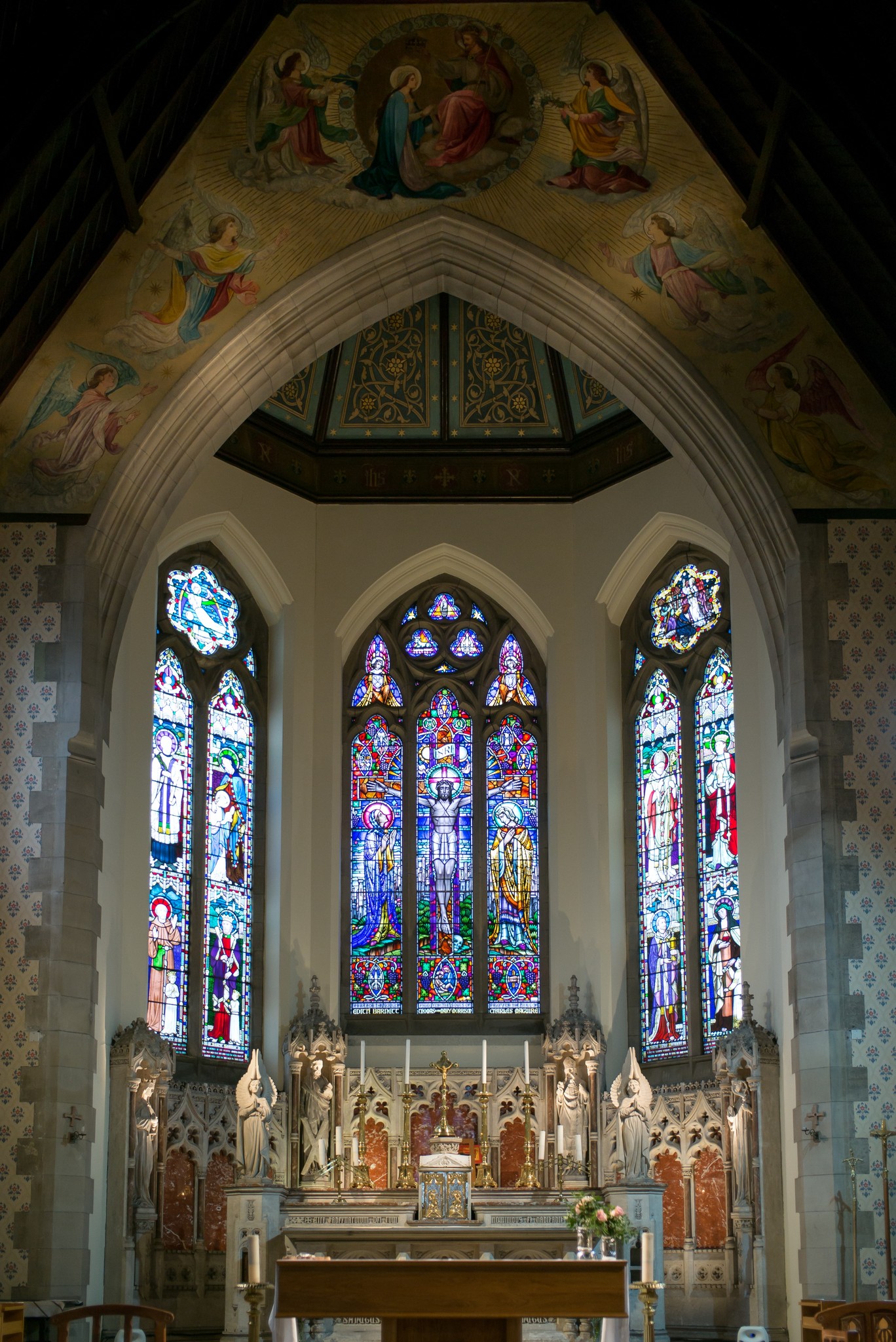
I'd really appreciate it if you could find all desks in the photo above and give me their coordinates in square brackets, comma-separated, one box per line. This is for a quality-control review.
[276, 1258, 629, 1342]
[0, 1302, 27, 1342]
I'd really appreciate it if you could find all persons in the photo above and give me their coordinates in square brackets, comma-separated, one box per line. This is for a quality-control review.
[300, 1059, 333, 1175]
[235, 1079, 273, 1179]
[436, 1064, 452, 1089]
[726, 1075, 755, 1206]
[616, 1079, 652, 1179]
[133, 1078, 158, 1208]
[556, 1056, 590, 1180]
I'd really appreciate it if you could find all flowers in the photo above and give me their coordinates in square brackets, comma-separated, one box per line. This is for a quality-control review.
[561, 1190, 642, 1244]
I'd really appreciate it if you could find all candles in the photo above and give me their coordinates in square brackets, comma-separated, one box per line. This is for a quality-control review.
[524, 1040, 529, 1084]
[575, 1133, 582, 1161]
[352, 1137, 359, 1166]
[360, 1040, 365, 1085]
[336, 1126, 341, 1155]
[642, 1232, 653, 1282]
[557, 1124, 563, 1154]
[482, 1039, 486, 1084]
[405, 1038, 410, 1084]
[247, 1234, 260, 1283]
[318, 1138, 325, 1165]
[81, 1125, 85, 1132]
[538, 1130, 546, 1160]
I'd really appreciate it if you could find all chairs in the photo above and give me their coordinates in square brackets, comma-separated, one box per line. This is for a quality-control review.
[814, 1300, 896, 1342]
[51, 1304, 174, 1342]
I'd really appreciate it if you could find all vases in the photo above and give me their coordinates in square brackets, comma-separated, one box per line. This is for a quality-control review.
[576, 1226, 616, 1261]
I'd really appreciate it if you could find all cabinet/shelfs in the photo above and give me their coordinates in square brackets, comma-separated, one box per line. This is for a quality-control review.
[798, 1299, 846, 1342]
